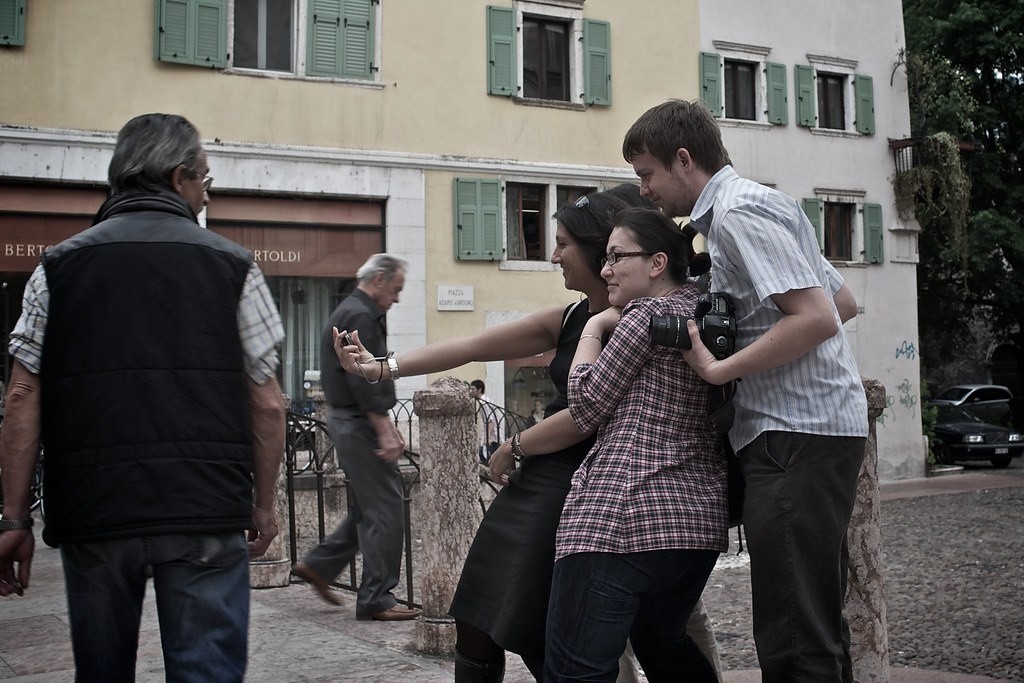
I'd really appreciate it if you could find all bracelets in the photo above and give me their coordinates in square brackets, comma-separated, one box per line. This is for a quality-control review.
[387, 358, 399, 380]
[0, 516, 34, 533]
[511, 434, 524, 462]
[515, 432, 529, 457]
[580, 335, 601, 344]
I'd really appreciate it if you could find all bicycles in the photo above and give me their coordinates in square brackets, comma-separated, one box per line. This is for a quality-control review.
[286, 413, 315, 474]
[29, 445, 47, 524]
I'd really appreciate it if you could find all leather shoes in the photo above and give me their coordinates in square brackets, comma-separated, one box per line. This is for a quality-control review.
[295, 563, 343, 606]
[357, 601, 421, 620]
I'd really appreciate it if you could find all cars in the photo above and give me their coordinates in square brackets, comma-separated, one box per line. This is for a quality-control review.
[929, 385, 1013, 420]
[922, 401, 1024, 469]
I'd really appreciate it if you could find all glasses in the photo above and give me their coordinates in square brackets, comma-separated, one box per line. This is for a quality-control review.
[601, 251, 651, 266]
[194, 171, 213, 191]
[575, 195, 604, 232]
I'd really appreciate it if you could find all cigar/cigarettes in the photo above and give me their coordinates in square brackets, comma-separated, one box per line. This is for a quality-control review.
[13, 582, 21, 587]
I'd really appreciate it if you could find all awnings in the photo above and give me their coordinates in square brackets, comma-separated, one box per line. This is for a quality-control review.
[1, 185, 107, 274]
[205, 196, 384, 278]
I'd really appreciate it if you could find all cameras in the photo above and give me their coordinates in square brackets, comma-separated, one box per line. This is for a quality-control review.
[342, 332, 354, 346]
[648, 293, 737, 360]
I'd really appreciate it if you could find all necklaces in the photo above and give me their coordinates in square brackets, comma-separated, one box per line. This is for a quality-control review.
[662, 287, 678, 297]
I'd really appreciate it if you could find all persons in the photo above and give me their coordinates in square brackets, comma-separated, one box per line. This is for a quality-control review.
[333, 183, 728, 683]
[0, 114, 287, 682]
[623, 100, 867, 683]
[294, 252, 421, 621]
